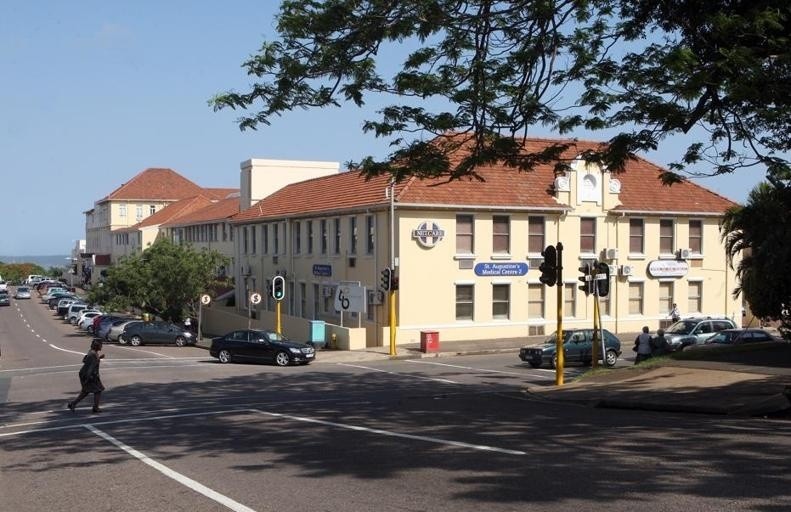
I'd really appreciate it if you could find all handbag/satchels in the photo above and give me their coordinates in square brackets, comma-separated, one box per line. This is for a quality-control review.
[82, 354, 90, 363]
[632, 336, 640, 352]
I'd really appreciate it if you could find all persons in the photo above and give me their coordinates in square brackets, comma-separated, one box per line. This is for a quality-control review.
[633, 326, 652, 364]
[653, 329, 670, 356]
[67, 338, 106, 414]
[668, 303, 680, 323]
[183, 317, 192, 331]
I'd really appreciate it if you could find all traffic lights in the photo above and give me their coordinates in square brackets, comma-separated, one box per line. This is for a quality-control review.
[391, 277, 399, 290]
[578, 262, 591, 296]
[578, 263, 593, 297]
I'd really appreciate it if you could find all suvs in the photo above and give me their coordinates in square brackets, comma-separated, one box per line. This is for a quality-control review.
[651, 317, 737, 351]
[77, 312, 103, 328]
[37, 282, 71, 295]
[0, 281, 7, 292]
[49, 293, 76, 310]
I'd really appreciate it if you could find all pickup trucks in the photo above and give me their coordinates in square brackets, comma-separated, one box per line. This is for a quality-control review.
[67, 304, 92, 324]
[57, 299, 80, 319]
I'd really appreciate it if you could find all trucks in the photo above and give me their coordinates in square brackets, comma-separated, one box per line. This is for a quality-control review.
[21, 275, 42, 285]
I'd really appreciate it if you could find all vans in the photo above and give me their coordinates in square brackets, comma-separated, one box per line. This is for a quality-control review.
[76, 309, 99, 326]
[46, 287, 67, 295]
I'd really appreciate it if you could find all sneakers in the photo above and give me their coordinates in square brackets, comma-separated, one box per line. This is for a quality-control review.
[67, 402, 79, 412]
[92, 405, 103, 413]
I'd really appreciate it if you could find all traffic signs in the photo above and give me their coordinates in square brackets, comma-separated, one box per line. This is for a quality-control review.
[585, 272, 607, 283]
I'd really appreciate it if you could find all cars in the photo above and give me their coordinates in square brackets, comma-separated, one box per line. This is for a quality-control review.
[0, 294, 10, 307]
[210, 329, 315, 366]
[93, 315, 115, 336]
[42, 291, 76, 304]
[29, 279, 65, 289]
[108, 319, 144, 345]
[122, 321, 196, 347]
[519, 328, 622, 369]
[98, 317, 130, 342]
[16, 287, 31, 299]
[683, 328, 791, 352]
[81, 315, 101, 335]
